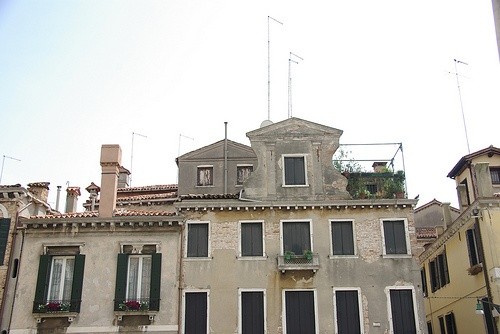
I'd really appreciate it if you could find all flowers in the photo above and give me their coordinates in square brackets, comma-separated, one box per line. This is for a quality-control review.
[46, 302, 61, 309]
[125, 301, 140, 310]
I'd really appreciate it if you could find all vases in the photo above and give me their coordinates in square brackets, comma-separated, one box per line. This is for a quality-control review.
[47, 308, 59, 314]
[127, 308, 138, 311]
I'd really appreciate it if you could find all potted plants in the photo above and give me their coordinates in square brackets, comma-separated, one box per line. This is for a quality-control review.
[285, 251, 294, 259]
[305, 251, 313, 259]
[395, 186, 405, 199]
